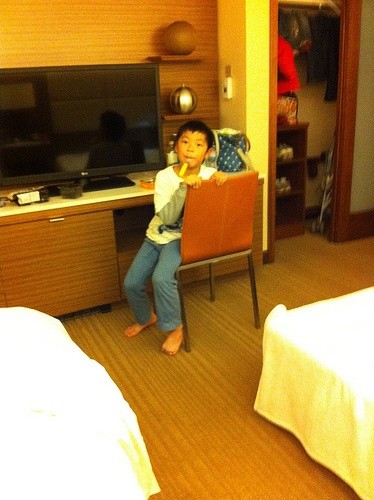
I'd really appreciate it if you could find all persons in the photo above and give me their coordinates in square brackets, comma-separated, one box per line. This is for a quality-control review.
[123, 122, 227, 356]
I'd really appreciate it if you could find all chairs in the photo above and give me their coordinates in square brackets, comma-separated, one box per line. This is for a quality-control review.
[178, 171, 259, 351]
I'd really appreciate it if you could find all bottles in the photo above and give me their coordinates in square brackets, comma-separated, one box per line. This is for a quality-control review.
[164, 141, 178, 167]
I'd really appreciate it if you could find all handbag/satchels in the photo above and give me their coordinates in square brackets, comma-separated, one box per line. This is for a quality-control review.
[277, 91, 299, 127]
[279, 10, 313, 56]
[211, 128, 251, 174]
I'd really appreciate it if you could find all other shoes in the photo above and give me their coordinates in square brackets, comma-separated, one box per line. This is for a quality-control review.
[277, 176, 291, 193]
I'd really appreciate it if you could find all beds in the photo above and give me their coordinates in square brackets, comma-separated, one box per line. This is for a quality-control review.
[253, 287, 374, 500]
[0, 299, 161, 499]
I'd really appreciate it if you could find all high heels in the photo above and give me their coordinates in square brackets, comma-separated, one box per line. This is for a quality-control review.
[277, 143, 293, 162]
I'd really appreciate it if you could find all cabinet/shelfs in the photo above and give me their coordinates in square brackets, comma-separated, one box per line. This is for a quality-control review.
[275, 125, 309, 239]
[151, 55, 210, 121]
[0, 175, 262, 312]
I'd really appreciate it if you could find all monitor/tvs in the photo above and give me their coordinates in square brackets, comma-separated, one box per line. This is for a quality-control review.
[0, 61, 167, 198]
